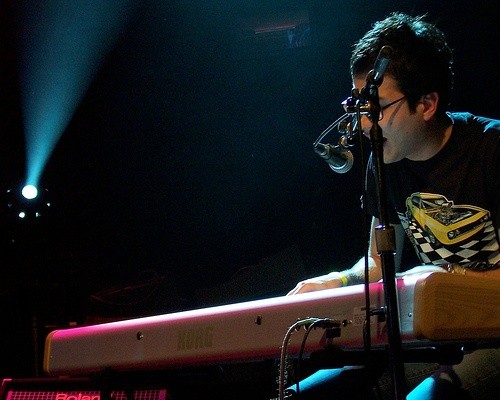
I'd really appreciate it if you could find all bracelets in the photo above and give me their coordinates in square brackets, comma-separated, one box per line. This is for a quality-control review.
[330, 271, 349, 287]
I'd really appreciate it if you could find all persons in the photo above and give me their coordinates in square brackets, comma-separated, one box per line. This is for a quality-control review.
[283, 11, 500, 400]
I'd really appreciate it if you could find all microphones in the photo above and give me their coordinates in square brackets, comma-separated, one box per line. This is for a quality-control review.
[313, 143, 354, 173]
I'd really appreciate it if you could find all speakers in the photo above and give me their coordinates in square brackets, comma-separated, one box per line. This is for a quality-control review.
[0, 377, 171, 400]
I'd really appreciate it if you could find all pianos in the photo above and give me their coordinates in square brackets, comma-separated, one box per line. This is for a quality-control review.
[43, 265, 499, 376]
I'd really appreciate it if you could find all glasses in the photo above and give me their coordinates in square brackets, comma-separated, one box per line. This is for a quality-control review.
[379, 96, 406, 121]
